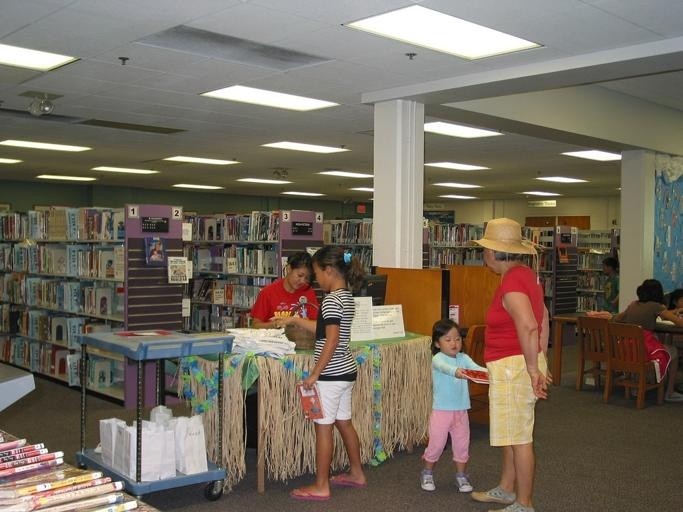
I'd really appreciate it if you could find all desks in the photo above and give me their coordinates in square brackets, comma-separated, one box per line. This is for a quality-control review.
[186, 325, 433, 498]
[1, 422, 169, 512]
[551, 311, 683, 390]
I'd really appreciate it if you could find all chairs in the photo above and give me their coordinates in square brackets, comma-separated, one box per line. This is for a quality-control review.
[604, 320, 667, 410]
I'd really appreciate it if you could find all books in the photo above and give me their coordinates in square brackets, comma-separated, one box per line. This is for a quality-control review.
[1, 205, 192, 391]
[460, 367, 489, 385]
[180, 210, 323, 333]
[574, 250, 606, 313]
[323, 217, 485, 341]
[0, 434, 138, 512]
[294, 379, 325, 420]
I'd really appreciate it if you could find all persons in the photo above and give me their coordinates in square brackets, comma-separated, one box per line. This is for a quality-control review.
[613, 277, 683, 404]
[601, 257, 620, 312]
[666, 289, 683, 317]
[149, 240, 163, 258]
[465, 216, 554, 512]
[271, 245, 369, 499]
[252, 252, 322, 327]
[418, 319, 488, 494]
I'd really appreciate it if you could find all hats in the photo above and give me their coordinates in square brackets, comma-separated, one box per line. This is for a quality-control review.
[468, 218, 538, 257]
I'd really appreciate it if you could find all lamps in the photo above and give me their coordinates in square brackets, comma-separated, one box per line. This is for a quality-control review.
[23, 87, 57, 118]
[272, 166, 292, 180]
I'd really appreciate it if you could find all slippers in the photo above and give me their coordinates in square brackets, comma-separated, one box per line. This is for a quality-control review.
[289, 487, 330, 501]
[329, 474, 367, 489]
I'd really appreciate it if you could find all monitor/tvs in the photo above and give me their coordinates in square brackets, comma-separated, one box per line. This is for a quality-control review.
[356, 273, 388, 306]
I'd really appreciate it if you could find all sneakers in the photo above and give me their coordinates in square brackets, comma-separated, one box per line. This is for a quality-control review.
[487, 500, 534, 512]
[418, 469, 435, 491]
[471, 486, 516, 505]
[454, 473, 472, 493]
[663, 392, 682, 402]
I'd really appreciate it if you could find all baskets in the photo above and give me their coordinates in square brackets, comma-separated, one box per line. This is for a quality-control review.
[284, 302, 319, 350]
